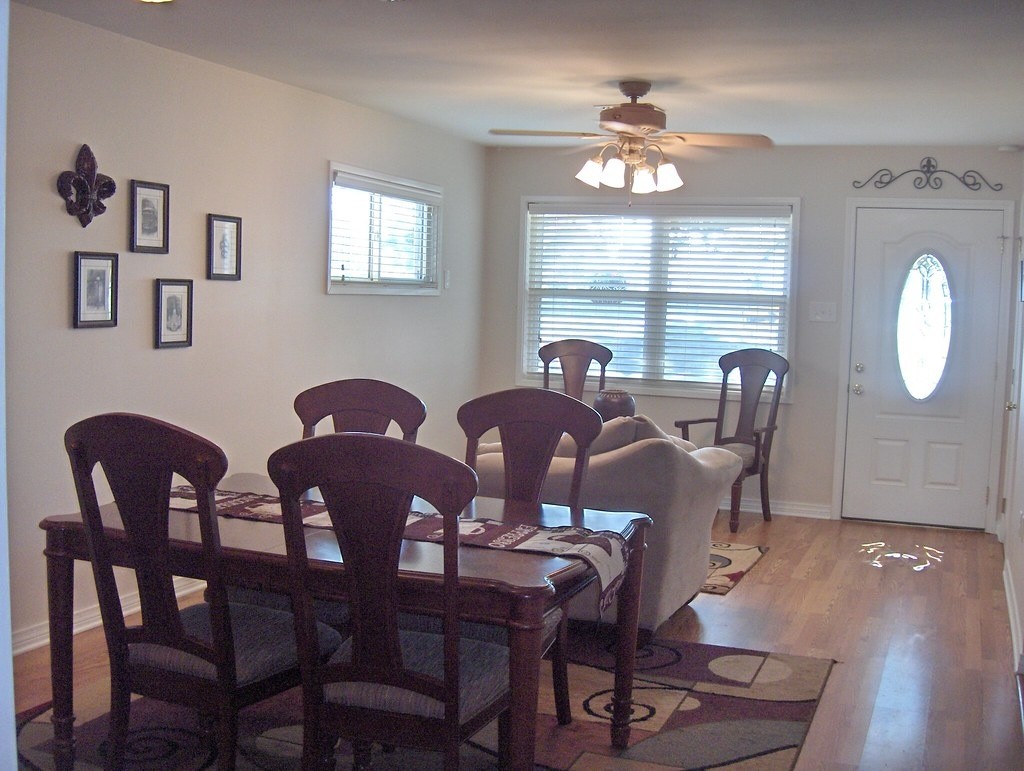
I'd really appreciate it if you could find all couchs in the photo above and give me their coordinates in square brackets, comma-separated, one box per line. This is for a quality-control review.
[474, 420, 743, 633]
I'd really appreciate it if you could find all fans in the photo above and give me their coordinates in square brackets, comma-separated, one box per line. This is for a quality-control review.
[492, 80, 772, 151]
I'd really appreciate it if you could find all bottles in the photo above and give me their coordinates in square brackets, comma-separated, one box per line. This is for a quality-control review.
[593, 389, 636, 422]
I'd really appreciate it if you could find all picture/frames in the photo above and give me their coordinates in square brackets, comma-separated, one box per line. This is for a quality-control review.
[130, 179, 170, 255]
[206, 213, 242, 280]
[74, 251, 117, 328]
[155, 278, 193, 348]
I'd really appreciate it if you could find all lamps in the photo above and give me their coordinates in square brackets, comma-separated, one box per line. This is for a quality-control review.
[575, 141, 684, 194]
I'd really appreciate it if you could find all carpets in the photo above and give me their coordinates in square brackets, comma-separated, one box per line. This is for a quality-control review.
[18, 638, 837, 771]
[701, 539, 770, 597]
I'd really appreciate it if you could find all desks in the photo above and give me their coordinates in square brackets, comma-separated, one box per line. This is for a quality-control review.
[44, 474, 654, 770]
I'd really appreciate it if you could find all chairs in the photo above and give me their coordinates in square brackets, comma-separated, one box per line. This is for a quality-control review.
[267, 431, 510, 770]
[673, 349, 790, 536]
[399, 387, 603, 725]
[539, 338, 614, 401]
[203, 378, 427, 626]
[65, 411, 343, 771]
